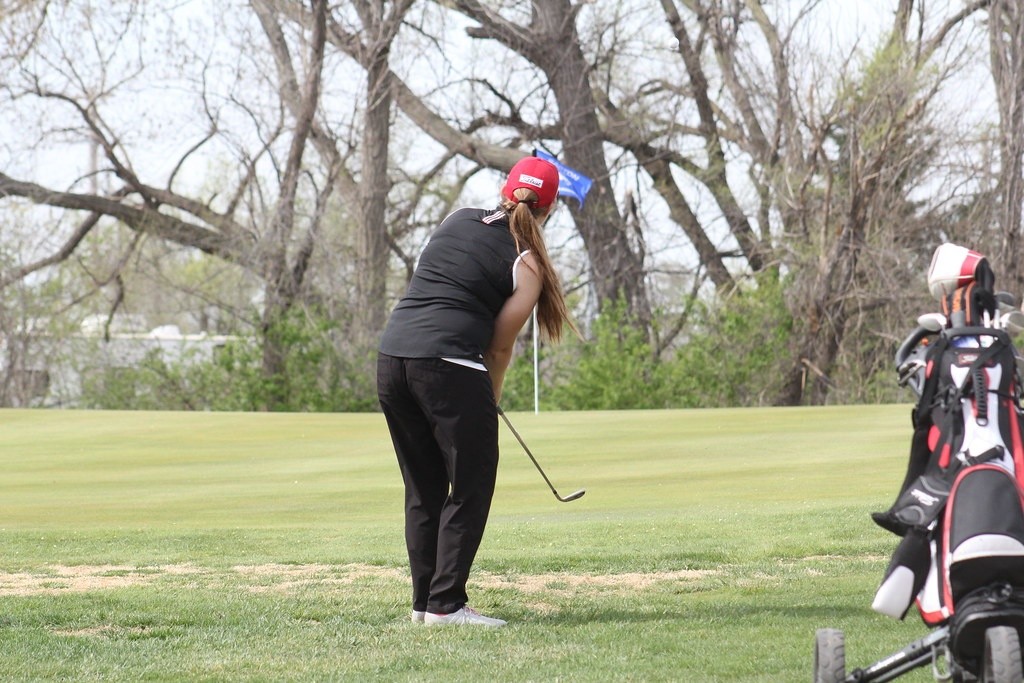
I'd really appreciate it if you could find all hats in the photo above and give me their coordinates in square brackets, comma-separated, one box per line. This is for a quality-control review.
[502, 156, 559, 209]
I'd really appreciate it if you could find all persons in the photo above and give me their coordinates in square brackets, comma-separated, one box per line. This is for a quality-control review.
[376, 156, 582, 630]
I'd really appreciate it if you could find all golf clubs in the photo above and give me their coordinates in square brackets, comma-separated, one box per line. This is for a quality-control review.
[917, 292, 1024, 332]
[497, 406, 586, 503]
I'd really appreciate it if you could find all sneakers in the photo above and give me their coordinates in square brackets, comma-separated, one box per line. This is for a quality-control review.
[425, 604, 506, 627]
[411, 609, 426, 623]
